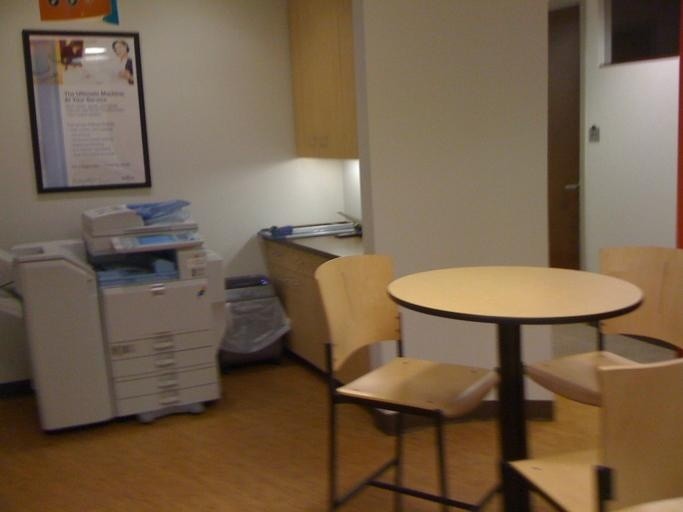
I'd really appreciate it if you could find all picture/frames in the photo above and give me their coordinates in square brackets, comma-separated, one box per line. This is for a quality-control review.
[22, 28, 154, 195]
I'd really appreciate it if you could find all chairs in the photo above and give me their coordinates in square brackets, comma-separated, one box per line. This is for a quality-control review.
[509, 358, 683, 512]
[526, 242, 683, 404]
[313, 255, 496, 512]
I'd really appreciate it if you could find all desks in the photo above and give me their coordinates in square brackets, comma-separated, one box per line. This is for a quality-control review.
[387, 265, 644, 512]
[258, 231, 369, 382]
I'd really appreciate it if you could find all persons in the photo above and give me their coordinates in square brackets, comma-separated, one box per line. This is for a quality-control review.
[112, 40, 134, 85]
[61, 39, 83, 70]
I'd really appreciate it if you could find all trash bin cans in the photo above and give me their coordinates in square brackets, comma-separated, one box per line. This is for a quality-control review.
[216, 275, 292, 373]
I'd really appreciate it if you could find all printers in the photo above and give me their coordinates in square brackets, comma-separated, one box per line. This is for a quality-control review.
[0, 199, 227, 431]
